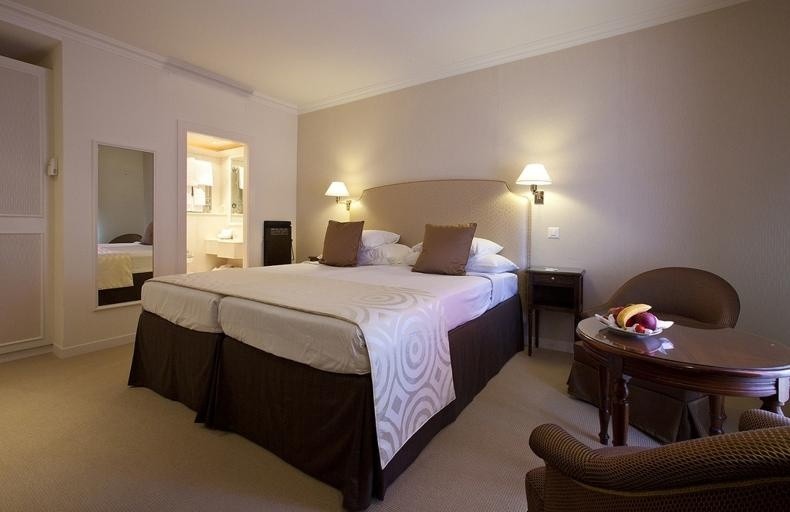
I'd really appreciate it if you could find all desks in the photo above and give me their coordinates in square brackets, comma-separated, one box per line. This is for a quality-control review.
[574, 312, 789, 446]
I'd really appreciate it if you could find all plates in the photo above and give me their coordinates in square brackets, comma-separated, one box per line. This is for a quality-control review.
[594, 312, 675, 338]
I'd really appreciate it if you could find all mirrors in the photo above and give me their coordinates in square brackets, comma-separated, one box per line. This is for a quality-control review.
[228, 154, 246, 216]
[92, 141, 161, 313]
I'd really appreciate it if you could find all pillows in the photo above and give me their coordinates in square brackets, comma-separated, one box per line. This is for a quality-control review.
[318, 219, 365, 267]
[359, 244, 410, 266]
[140, 221, 153, 244]
[317, 228, 402, 259]
[414, 253, 520, 274]
[409, 232, 503, 258]
[410, 222, 478, 278]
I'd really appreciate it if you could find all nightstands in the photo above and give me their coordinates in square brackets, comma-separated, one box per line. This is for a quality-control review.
[523, 263, 586, 358]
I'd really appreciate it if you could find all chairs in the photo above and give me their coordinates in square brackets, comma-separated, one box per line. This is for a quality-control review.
[524, 406, 790, 511]
[564, 267, 746, 445]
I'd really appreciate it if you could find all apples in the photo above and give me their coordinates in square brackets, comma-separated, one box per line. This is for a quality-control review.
[626, 311, 656, 330]
[608, 306, 624, 319]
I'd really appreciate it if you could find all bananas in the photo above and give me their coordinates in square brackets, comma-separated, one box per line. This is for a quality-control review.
[616, 304, 652, 330]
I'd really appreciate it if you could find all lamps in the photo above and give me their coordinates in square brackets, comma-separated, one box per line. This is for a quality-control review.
[323, 180, 352, 212]
[514, 162, 554, 206]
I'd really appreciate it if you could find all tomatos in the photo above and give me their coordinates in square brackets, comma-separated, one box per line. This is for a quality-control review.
[635, 326, 646, 333]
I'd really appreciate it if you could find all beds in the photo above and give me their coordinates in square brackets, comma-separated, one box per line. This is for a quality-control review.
[96, 241, 153, 306]
[127, 178, 531, 512]
